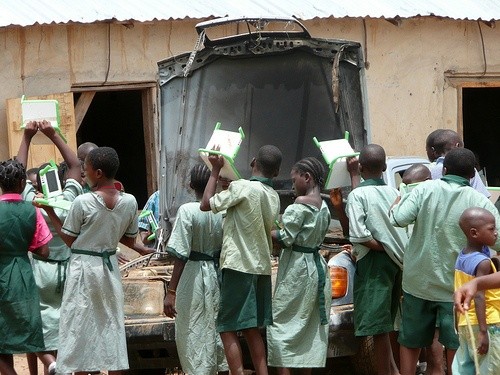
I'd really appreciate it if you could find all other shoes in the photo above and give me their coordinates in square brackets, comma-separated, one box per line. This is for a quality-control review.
[50, 367, 57, 375]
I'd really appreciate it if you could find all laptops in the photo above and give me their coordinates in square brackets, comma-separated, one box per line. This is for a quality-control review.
[34, 160, 72, 212]
[313, 130, 361, 190]
[138, 210, 157, 240]
[484, 187, 500, 205]
[400, 183, 419, 198]
[20, 95, 68, 145]
[197, 122, 245, 181]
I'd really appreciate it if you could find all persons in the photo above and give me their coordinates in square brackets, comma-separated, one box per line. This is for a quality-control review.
[33, 147, 139, 375]
[137, 190, 160, 253]
[0, 119, 125, 375]
[200, 145, 281, 375]
[163, 162, 231, 375]
[331, 130, 500, 375]
[267, 157, 331, 375]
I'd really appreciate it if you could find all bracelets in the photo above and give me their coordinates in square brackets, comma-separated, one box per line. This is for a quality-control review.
[168, 289, 176, 293]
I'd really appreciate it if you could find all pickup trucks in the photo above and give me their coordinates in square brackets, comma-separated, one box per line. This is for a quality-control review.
[34, 156, 431, 375]
